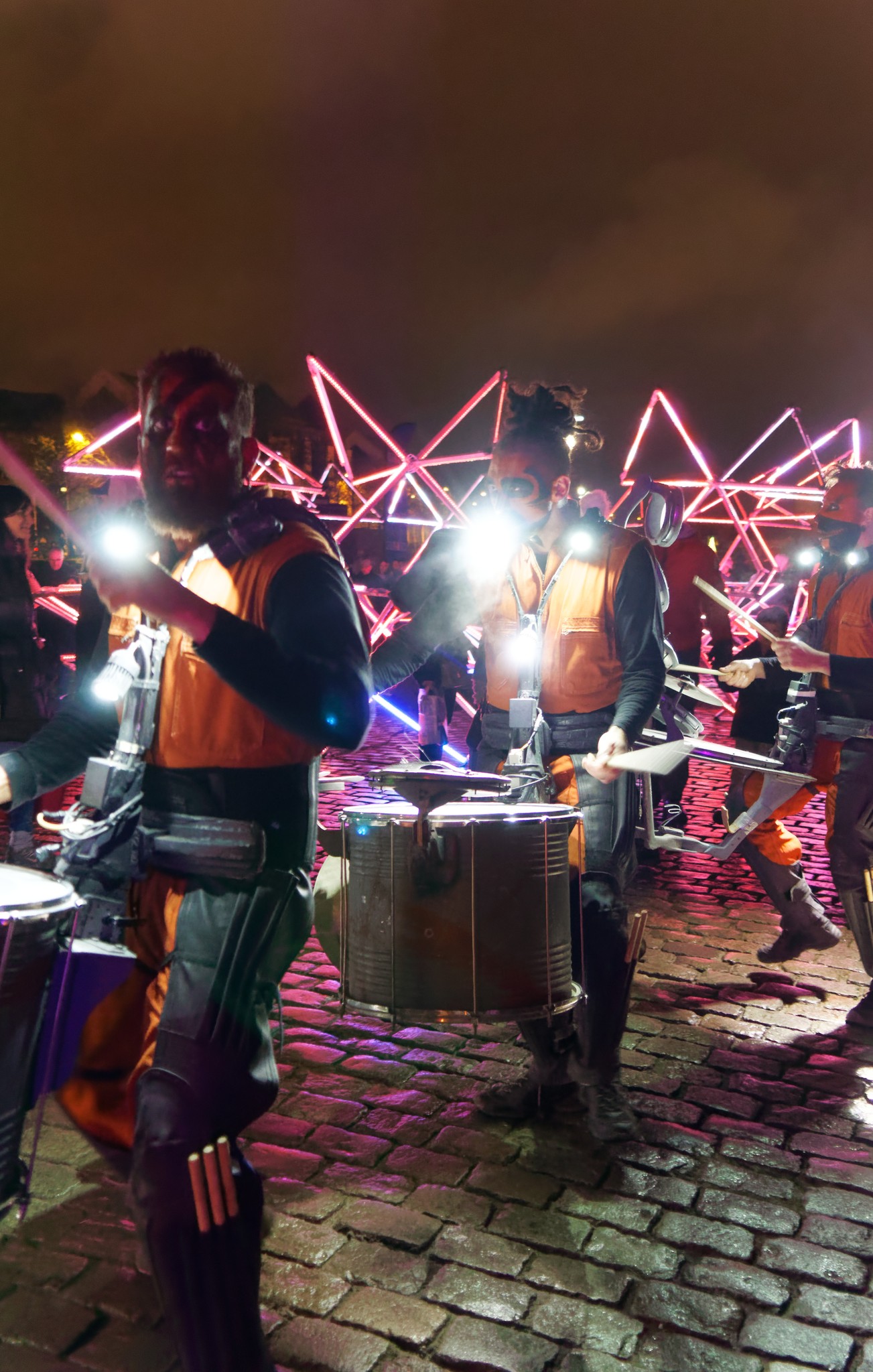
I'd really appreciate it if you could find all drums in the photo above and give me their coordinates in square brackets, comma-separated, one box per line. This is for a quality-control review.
[0, 858, 87, 1219]
[312, 761, 590, 1036]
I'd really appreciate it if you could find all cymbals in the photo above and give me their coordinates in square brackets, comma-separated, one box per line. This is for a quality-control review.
[662, 672, 726, 707]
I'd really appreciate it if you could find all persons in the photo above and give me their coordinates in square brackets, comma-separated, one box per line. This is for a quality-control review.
[387, 523, 486, 770]
[715, 466, 873, 1030]
[32, 548, 84, 686]
[363, 383, 671, 1143]
[618, 481, 740, 830]
[0, 480, 81, 871]
[707, 602, 807, 822]
[0, 344, 377, 1371]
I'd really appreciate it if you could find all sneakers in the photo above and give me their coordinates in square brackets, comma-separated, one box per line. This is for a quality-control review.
[661, 804, 688, 830]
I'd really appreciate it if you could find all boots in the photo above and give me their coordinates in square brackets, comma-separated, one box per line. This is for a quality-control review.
[569, 871, 642, 1143]
[837, 865, 873, 1026]
[133, 1129, 277, 1371]
[735, 836, 843, 964]
[474, 1008, 580, 1121]
